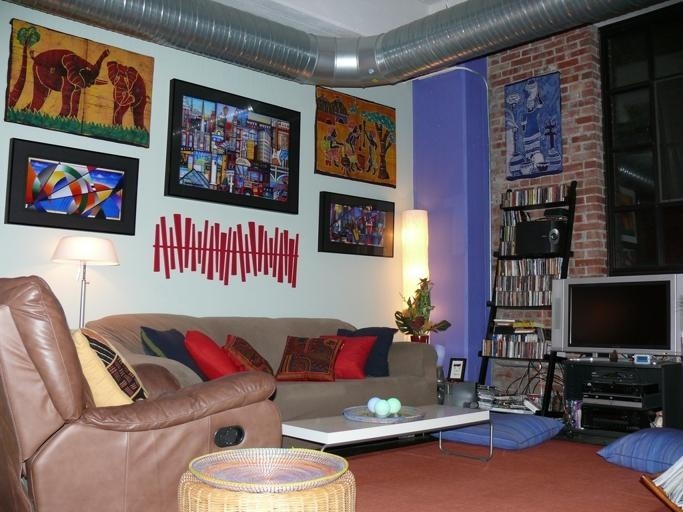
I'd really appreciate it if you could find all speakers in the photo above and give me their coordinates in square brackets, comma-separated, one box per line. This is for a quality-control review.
[517, 220, 567, 256]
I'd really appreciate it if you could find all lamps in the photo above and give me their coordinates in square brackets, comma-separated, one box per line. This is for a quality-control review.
[50, 237, 118, 328]
[402, 208, 431, 344]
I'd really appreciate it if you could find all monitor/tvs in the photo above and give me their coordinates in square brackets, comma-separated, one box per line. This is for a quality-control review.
[550, 274, 682, 363]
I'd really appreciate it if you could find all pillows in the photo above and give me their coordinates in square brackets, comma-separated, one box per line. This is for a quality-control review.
[596, 426, 683, 475]
[183, 330, 238, 379]
[432, 413, 567, 450]
[77, 329, 149, 407]
[223, 334, 276, 402]
[337, 326, 396, 377]
[274, 336, 344, 382]
[320, 335, 379, 381]
[142, 326, 203, 381]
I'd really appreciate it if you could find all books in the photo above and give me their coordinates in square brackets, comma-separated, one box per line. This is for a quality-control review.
[495, 257, 562, 306]
[499, 211, 530, 256]
[501, 184, 569, 208]
[481, 318, 551, 360]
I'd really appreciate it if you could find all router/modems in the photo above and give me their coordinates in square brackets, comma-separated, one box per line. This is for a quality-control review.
[523, 398, 540, 412]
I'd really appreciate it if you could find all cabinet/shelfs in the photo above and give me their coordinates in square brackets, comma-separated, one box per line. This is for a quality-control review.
[478, 178, 579, 411]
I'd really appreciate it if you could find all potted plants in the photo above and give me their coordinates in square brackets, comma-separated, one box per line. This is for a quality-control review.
[396, 278, 451, 341]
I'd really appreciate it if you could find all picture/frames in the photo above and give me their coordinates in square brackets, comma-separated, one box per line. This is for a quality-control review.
[319, 191, 396, 258]
[447, 357, 465, 381]
[4, 17, 154, 150]
[502, 72, 563, 180]
[165, 78, 300, 217]
[314, 82, 400, 188]
[4, 138, 140, 235]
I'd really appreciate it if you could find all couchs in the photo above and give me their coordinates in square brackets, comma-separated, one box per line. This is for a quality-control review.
[0, 276, 281, 507]
[86, 314, 439, 424]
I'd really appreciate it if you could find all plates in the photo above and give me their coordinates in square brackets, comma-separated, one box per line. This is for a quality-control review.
[342, 405, 426, 424]
